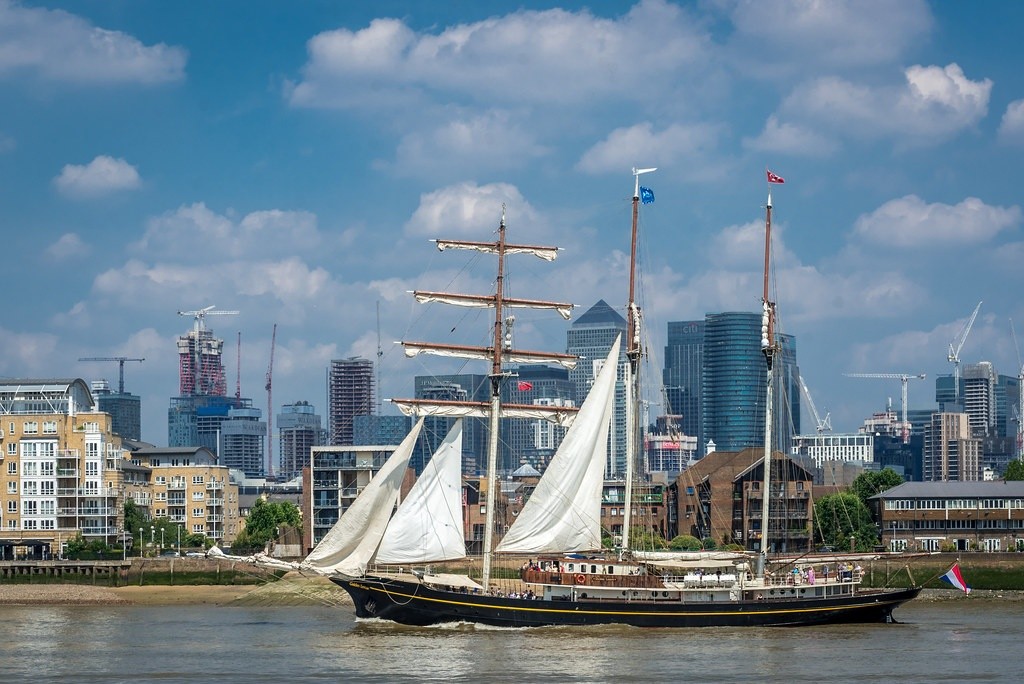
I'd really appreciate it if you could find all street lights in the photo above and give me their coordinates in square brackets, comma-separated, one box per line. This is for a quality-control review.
[151, 526, 155, 542]
[139, 528, 143, 558]
[892, 520, 897, 538]
[161, 528, 164, 548]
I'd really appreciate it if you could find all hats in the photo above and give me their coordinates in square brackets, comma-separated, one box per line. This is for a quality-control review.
[844, 563, 847, 565]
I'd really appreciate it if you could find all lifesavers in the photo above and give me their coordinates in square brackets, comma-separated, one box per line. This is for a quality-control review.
[577, 574, 585, 582]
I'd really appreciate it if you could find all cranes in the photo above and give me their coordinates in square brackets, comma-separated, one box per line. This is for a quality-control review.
[947, 301, 985, 414]
[797, 373, 834, 434]
[264, 324, 278, 474]
[235, 330, 243, 408]
[78, 357, 145, 395]
[175, 303, 242, 395]
[838, 371, 927, 447]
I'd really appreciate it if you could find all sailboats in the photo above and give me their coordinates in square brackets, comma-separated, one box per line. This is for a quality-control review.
[207, 166, 962, 630]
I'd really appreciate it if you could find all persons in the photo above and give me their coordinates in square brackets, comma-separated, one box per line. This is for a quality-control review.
[630, 563, 862, 586]
[444, 558, 566, 600]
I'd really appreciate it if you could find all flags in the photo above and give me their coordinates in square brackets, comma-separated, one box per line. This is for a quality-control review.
[640, 187, 654, 204]
[938, 564, 972, 596]
[767, 171, 784, 184]
